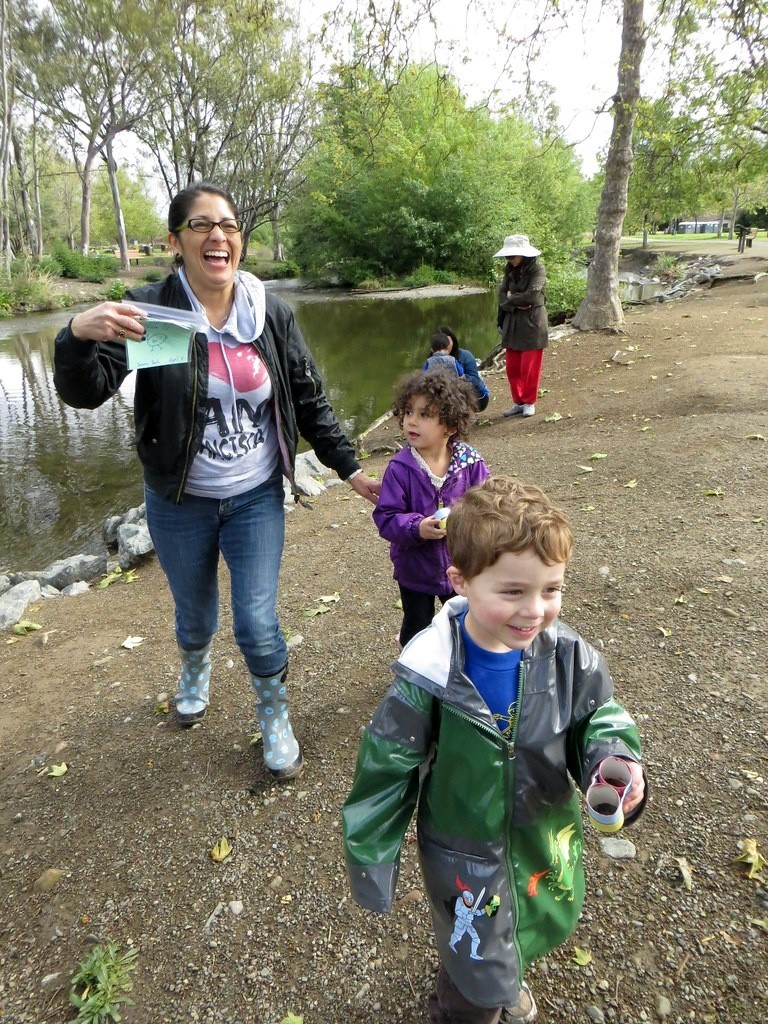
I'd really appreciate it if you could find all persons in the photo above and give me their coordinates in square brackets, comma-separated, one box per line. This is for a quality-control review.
[369, 370, 494, 652]
[425, 333, 465, 386]
[53, 184, 383, 782]
[492, 234, 548, 416]
[429, 324, 490, 425]
[342, 472, 649, 1024]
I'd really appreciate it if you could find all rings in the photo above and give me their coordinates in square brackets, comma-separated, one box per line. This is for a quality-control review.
[117, 329, 126, 337]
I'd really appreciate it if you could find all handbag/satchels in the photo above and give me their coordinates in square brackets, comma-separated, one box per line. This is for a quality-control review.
[497, 305, 506, 329]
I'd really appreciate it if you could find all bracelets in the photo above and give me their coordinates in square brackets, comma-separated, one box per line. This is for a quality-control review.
[346, 468, 363, 484]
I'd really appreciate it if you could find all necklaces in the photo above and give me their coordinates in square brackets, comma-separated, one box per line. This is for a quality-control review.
[210, 314, 228, 329]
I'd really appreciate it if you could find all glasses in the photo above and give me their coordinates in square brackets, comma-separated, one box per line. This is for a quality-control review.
[505, 256, 515, 259]
[173, 218, 243, 233]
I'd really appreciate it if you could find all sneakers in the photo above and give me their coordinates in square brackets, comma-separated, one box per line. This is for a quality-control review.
[523, 403, 535, 416]
[504, 404, 523, 417]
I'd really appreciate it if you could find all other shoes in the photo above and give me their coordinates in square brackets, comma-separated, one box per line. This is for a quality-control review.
[502, 982, 538, 1024]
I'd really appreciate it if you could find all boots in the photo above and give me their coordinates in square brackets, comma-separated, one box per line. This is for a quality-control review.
[251, 661, 303, 780]
[176, 638, 213, 725]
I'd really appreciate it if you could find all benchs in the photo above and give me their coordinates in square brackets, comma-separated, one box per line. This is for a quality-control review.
[114, 250, 145, 266]
[745, 228, 757, 248]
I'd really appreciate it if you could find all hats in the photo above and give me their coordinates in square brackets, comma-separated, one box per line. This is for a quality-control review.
[494, 234, 541, 257]
